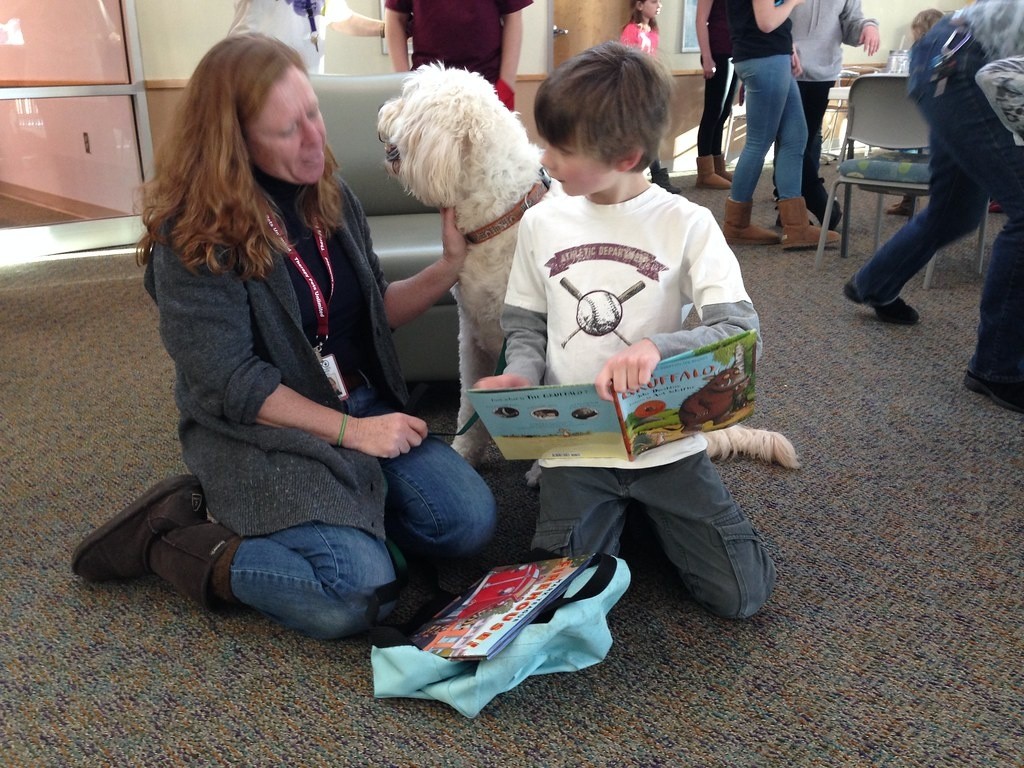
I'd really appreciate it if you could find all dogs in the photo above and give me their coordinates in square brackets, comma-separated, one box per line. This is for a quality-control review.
[374, 59, 802, 471]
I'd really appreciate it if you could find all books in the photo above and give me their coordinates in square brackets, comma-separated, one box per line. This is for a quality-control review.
[466, 326, 758, 461]
[408, 553, 597, 663]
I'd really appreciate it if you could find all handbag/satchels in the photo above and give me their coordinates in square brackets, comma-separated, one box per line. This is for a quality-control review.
[364, 545, 633, 719]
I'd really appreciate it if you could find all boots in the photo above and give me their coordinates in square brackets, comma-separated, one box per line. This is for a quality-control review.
[778, 196, 841, 249]
[723, 196, 782, 245]
[648, 168, 681, 194]
[69, 475, 237, 617]
[695, 151, 734, 189]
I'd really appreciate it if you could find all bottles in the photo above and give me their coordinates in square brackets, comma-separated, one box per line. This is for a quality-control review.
[885, 49, 909, 73]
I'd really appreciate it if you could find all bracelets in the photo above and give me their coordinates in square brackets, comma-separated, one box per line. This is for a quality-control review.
[338, 413, 349, 446]
[380, 21, 385, 39]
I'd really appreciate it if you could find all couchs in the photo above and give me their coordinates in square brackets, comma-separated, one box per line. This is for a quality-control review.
[304, 69, 465, 384]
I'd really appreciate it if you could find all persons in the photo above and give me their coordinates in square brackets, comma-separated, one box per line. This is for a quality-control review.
[844, 0, 1024, 413]
[72, 33, 498, 639]
[385, 0, 534, 111]
[328, 377, 342, 396]
[694, 0, 878, 249]
[474, 40, 776, 621]
[619, 0, 682, 194]
[885, 9, 948, 216]
[228, 0, 414, 76]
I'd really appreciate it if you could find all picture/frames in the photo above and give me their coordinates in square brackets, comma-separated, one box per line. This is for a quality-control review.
[680, 0, 708, 53]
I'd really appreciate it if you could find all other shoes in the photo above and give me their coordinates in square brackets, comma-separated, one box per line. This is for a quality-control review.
[843, 274, 919, 323]
[810, 199, 843, 231]
[963, 369, 1024, 414]
[885, 195, 912, 216]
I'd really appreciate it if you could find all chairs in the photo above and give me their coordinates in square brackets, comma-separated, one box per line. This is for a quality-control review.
[812, 75, 990, 291]
[722, 103, 748, 164]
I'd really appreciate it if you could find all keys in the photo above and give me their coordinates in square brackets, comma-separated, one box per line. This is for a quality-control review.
[929, 53, 957, 98]
